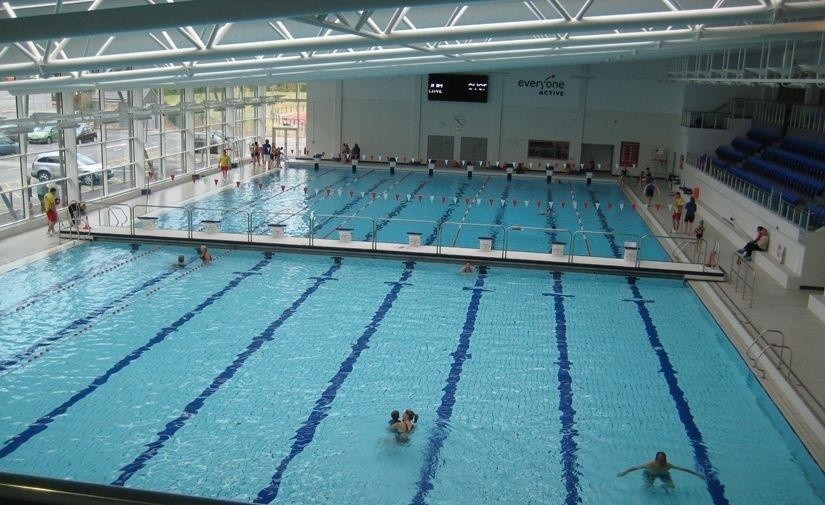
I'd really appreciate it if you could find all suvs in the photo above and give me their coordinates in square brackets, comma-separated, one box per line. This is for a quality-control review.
[30, 149, 115, 186]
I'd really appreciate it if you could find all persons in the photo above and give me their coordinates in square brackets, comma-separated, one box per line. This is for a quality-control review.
[250, 139, 284, 166]
[617, 451, 706, 496]
[218, 150, 231, 178]
[738, 226, 768, 260]
[671, 192, 697, 235]
[388, 408, 402, 426]
[199, 245, 211, 260]
[69, 201, 87, 225]
[640, 167, 651, 185]
[386, 409, 419, 446]
[44, 187, 60, 236]
[695, 220, 704, 239]
[342, 143, 361, 162]
[621, 165, 627, 178]
[177, 255, 185, 265]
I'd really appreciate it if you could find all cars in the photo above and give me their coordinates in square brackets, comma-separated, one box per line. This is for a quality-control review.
[0, 121, 95, 155]
[193, 129, 228, 155]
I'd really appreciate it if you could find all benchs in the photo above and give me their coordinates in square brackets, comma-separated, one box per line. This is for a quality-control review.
[700, 126, 825, 229]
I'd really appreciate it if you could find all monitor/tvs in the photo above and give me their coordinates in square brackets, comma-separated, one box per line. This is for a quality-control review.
[428, 74, 489, 104]
